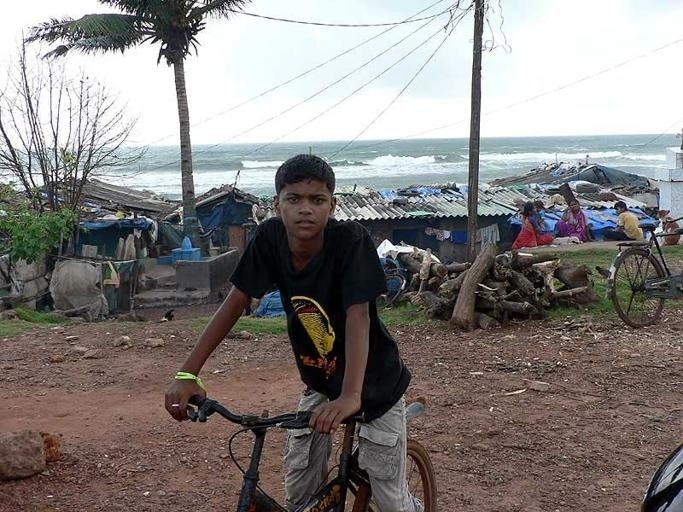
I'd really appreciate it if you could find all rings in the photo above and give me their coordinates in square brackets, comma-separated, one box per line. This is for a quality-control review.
[171, 403, 180, 407]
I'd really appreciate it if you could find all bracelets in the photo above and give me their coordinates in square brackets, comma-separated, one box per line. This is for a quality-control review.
[175, 375, 195, 381]
[176, 371, 196, 377]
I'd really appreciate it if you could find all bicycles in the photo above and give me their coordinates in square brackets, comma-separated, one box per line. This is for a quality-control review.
[605, 210, 683, 327]
[183, 395, 438, 511]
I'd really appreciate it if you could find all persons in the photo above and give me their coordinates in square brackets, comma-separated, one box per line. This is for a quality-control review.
[554, 199, 588, 243]
[510, 201, 553, 250]
[659, 210, 680, 246]
[163, 155, 427, 512]
[645, 210, 664, 248]
[601, 200, 643, 241]
[533, 199, 549, 230]
